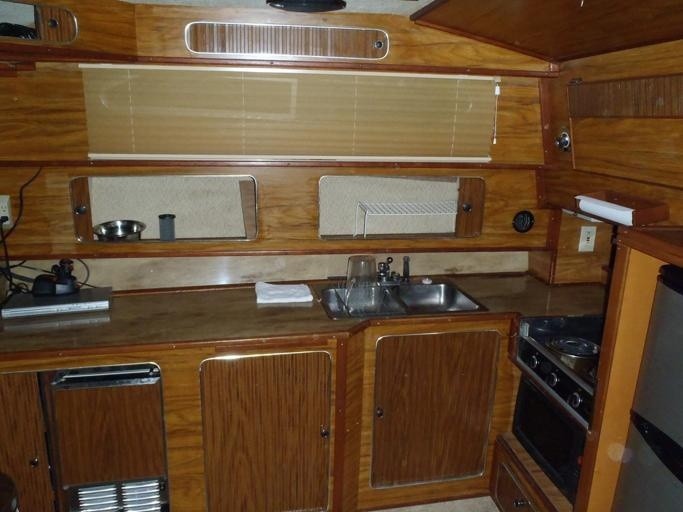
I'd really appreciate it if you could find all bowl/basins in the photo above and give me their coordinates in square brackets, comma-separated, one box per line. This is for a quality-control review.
[92, 220, 147, 241]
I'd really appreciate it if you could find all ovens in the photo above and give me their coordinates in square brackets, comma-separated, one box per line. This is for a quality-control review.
[511, 373, 588, 504]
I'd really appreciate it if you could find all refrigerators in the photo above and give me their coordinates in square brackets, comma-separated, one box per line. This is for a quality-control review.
[606, 265, 683, 512]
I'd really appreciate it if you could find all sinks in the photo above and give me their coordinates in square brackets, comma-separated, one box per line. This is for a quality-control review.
[308, 282, 407, 321]
[386, 281, 489, 316]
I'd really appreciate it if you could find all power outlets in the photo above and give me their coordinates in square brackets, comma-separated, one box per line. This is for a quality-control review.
[0, 194, 14, 231]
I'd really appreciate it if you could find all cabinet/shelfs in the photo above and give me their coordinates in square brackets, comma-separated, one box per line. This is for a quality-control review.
[0, 345, 197, 512]
[491, 432, 573, 512]
[195, 308, 520, 512]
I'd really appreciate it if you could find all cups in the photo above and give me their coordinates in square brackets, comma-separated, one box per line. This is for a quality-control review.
[345, 254, 378, 313]
[159, 214, 176, 241]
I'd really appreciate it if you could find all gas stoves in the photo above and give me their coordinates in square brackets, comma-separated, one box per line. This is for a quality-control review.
[515, 315, 606, 430]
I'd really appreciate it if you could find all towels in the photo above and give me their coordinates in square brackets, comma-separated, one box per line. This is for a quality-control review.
[252, 282, 314, 304]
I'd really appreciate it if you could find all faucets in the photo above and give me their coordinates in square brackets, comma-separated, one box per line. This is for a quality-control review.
[378, 256, 393, 279]
